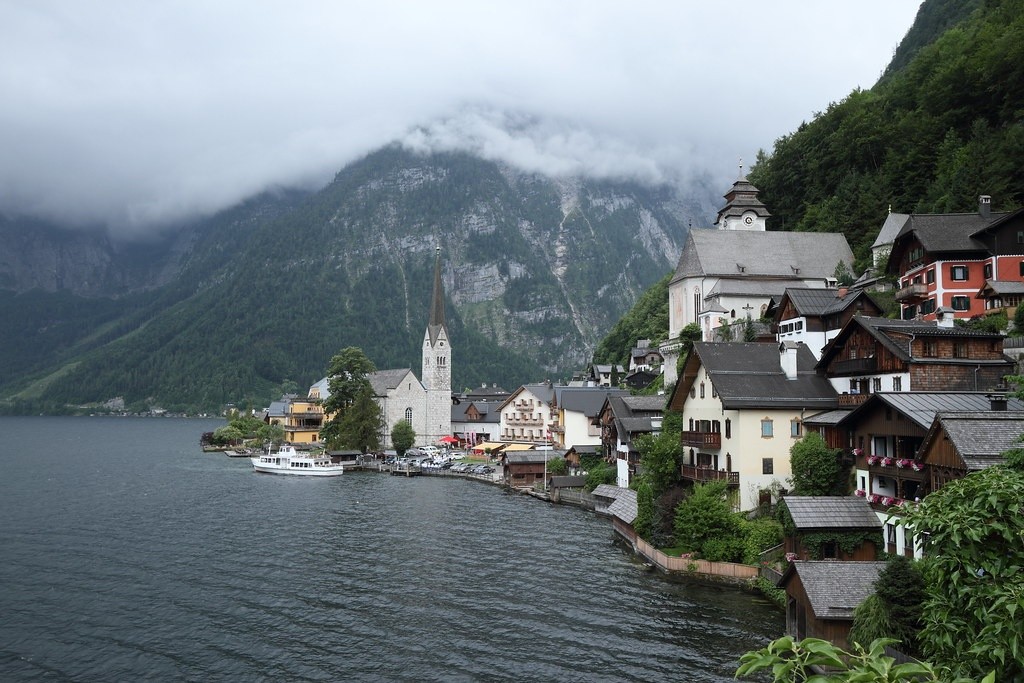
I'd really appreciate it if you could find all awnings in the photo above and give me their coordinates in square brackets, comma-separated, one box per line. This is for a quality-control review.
[470, 442, 505, 466]
[500, 444, 534, 451]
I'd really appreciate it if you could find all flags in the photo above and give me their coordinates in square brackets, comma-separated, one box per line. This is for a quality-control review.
[547, 430, 550, 440]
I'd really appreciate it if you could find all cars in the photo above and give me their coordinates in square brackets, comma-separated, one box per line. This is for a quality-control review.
[381, 445, 496, 475]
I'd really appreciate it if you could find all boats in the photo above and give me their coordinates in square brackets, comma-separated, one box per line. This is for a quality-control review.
[250, 445, 343, 476]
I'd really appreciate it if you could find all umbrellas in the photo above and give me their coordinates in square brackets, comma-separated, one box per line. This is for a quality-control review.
[441, 436, 458, 449]
